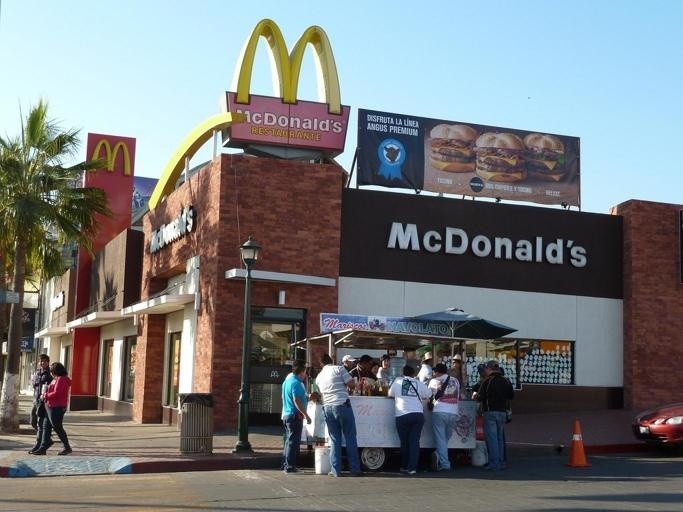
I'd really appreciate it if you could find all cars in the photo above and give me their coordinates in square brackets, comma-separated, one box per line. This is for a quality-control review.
[632, 401, 683, 451]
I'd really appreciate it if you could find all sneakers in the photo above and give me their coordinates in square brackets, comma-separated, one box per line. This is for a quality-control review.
[27, 438, 72, 455]
[279, 452, 506, 477]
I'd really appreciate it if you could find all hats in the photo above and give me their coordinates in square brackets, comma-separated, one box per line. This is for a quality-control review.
[422, 352, 432, 362]
[341, 354, 357, 363]
[448, 354, 461, 361]
[431, 364, 447, 373]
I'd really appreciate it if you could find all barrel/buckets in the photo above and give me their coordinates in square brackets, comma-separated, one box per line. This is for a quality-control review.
[389, 358, 406, 377]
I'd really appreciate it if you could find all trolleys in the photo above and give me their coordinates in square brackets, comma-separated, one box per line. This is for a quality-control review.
[290, 392, 476, 470]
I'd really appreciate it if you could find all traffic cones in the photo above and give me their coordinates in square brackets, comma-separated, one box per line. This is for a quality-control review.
[562, 420, 593, 468]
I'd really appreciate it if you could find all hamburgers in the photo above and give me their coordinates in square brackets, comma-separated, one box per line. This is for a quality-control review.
[429, 124, 477, 172]
[475, 132, 528, 183]
[523, 133, 567, 182]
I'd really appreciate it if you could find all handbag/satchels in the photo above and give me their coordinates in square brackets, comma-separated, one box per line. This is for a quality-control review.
[428, 396, 434, 410]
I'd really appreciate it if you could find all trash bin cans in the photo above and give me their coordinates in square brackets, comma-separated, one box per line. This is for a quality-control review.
[179, 392, 214, 454]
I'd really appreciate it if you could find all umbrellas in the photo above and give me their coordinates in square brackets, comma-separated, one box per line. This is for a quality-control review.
[399, 307, 519, 378]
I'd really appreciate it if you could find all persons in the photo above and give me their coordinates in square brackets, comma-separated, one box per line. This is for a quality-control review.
[282, 351, 515, 477]
[32, 362, 72, 455]
[28, 354, 54, 454]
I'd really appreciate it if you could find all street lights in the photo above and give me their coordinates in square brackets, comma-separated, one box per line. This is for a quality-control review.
[229, 234, 262, 457]
